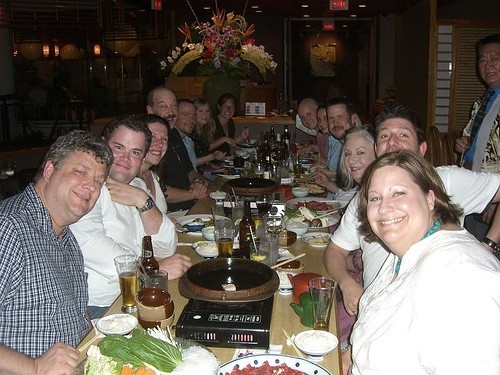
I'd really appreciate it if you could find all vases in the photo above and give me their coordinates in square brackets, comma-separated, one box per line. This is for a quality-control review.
[203, 76, 241, 112]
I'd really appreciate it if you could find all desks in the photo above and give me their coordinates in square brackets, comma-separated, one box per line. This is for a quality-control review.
[90, 115, 296, 145]
[74, 155, 342, 375]
[22, 101, 84, 141]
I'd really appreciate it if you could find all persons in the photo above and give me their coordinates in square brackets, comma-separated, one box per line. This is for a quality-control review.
[89, 77, 107, 112]
[129, 113, 170, 215]
[212, 92, 249, 157]
[0, 130, 115, 375]
[173, 99, 226, 188]
[314, 98, 359, 200]
[146, 87, 209, 216]
[322, 105, 500, 314]
[69, 116, 192, 323]
[187, 98, 236, 167]
[348, 149, 500, 374]
[335, 124, 377, 351]
[296, 98, 330, 168]
[456, 34, 500, 240]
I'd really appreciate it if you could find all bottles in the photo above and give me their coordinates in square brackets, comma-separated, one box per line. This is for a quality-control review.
[239, 200, 255, 250]
[138, 235, 159, 289]
[260, 125, 292, 179]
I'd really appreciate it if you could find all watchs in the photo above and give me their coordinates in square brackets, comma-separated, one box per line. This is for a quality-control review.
[136, 197, 154, 213]
[194, 178, 206, 187]
[481, 236, 500, 254]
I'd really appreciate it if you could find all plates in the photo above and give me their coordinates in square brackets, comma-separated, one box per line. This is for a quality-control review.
[216, 138, 260, 179]
[217, 353, 332, 375]
[286, 143, 343, 247]
[175, 214, 231, 235]
[196, 245, 218, 257]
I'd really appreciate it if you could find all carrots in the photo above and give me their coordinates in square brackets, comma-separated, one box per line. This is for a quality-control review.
[119, 365, 157, 375]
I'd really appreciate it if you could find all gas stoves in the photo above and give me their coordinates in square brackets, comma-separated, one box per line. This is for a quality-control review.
[171, 294, 273, 348]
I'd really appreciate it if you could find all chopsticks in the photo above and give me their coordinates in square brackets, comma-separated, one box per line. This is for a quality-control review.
[270, 253, 307, 269]
[282, 328, 305, 359]
[78, 335, 100, 351]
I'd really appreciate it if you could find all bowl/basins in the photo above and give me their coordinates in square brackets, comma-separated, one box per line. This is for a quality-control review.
[291, 187, 309, 197]
[278, 231, 296, 246]
[177, 258, 280, 304]
[73, 287, 220, 375]
[293, 329, 339, 360]
[286, 222, 310, 235]
[194, 177, 276, 258]
[275, 259, 304, 274]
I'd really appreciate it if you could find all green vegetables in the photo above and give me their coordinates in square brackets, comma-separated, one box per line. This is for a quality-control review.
[281, 205, 318, 219]
[99, 328, 185, 373]
[83, 345, 121, 375]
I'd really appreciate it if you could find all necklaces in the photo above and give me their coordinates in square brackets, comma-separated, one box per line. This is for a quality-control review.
[395, 218, 441, 271]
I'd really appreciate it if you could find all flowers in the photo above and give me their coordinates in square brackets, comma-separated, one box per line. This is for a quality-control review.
[158, 7, 278, 88]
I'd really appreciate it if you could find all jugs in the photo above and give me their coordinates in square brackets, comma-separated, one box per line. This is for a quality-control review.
[287, 273, 327, 327]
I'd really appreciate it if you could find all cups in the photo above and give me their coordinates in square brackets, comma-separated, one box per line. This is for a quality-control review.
[215, 229, 235, 257]
[114, 255, 168, 314]
[308, 277, 337, 332]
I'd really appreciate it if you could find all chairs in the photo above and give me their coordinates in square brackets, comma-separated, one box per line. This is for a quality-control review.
[428, 125, 461, 169]
